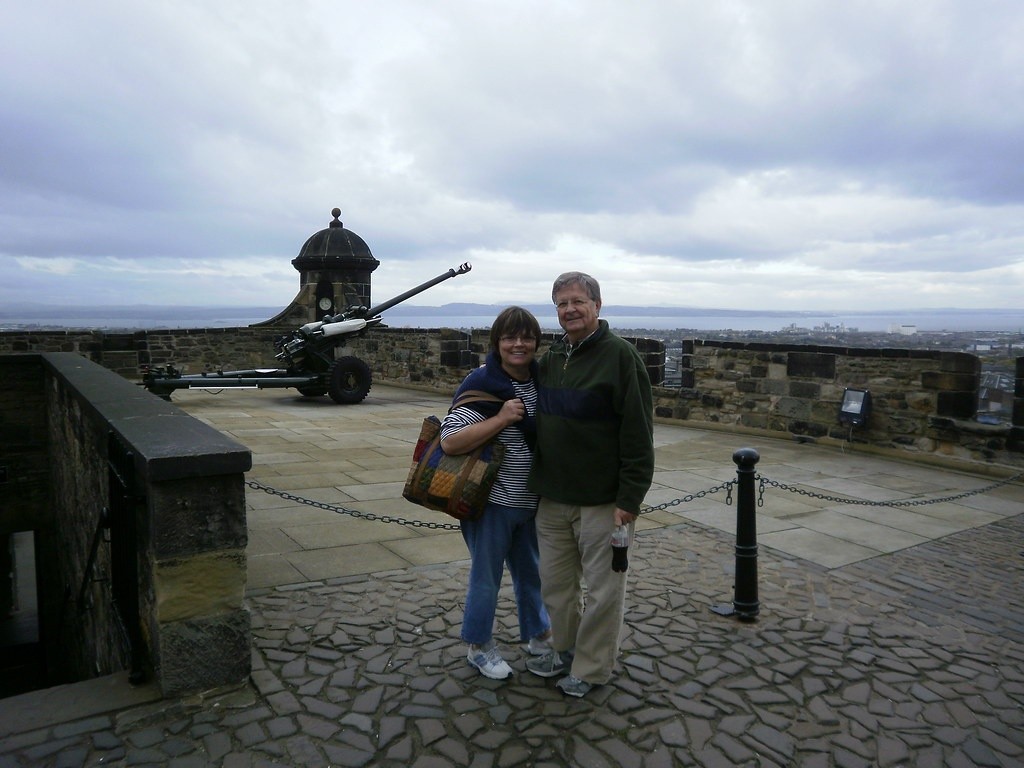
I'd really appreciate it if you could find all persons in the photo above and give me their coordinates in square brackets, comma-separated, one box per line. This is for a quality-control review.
[526, 272, 655, 696]
[439, 307, 563, 680]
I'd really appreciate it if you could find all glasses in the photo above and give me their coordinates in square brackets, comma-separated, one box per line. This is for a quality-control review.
[499, 334, 536, 343]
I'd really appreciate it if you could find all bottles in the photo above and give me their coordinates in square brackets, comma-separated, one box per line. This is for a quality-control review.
[611, 524, 629, 573]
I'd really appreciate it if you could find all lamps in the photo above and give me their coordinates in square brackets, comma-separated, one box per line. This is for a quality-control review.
[838, 388, 872, 426]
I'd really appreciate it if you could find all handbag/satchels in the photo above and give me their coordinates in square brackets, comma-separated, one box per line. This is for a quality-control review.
[403, 389, 508, 522]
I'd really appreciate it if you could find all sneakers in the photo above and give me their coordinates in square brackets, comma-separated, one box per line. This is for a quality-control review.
[555, 672, 599, 697]
[525, 652, 566, 677]
[527, 636, 553, 655]
[466, 642, 513, 679]
